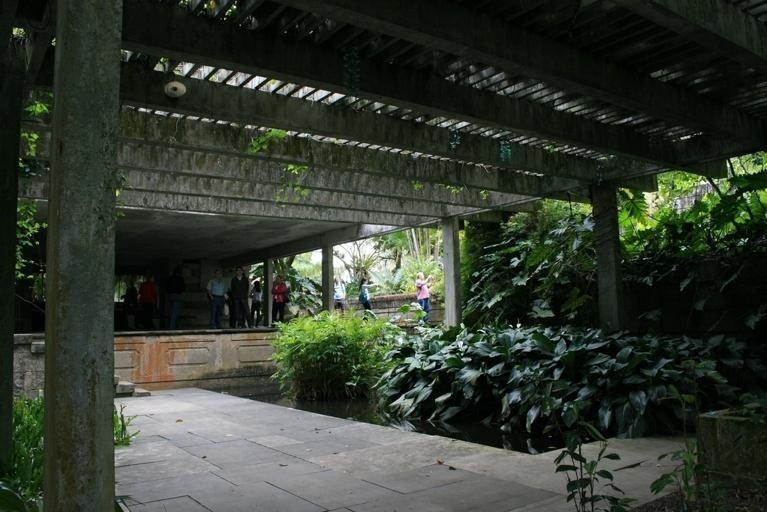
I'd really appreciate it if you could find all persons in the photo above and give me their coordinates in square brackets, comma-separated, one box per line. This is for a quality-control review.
[164, 263, 186, 330]
[415, 272, 434, 325]
[206, 266, 263, 329]
[120, 279, 138, 331]
[358, 277, 378, 321]
[138, 273, 157, 332]
[271, 274, 288, 328]
[333, 274, 346, 316]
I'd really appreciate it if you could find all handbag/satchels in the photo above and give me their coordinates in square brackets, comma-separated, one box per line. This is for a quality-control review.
[358, 292, 367, 305]
[284, 292, 290, 303]
